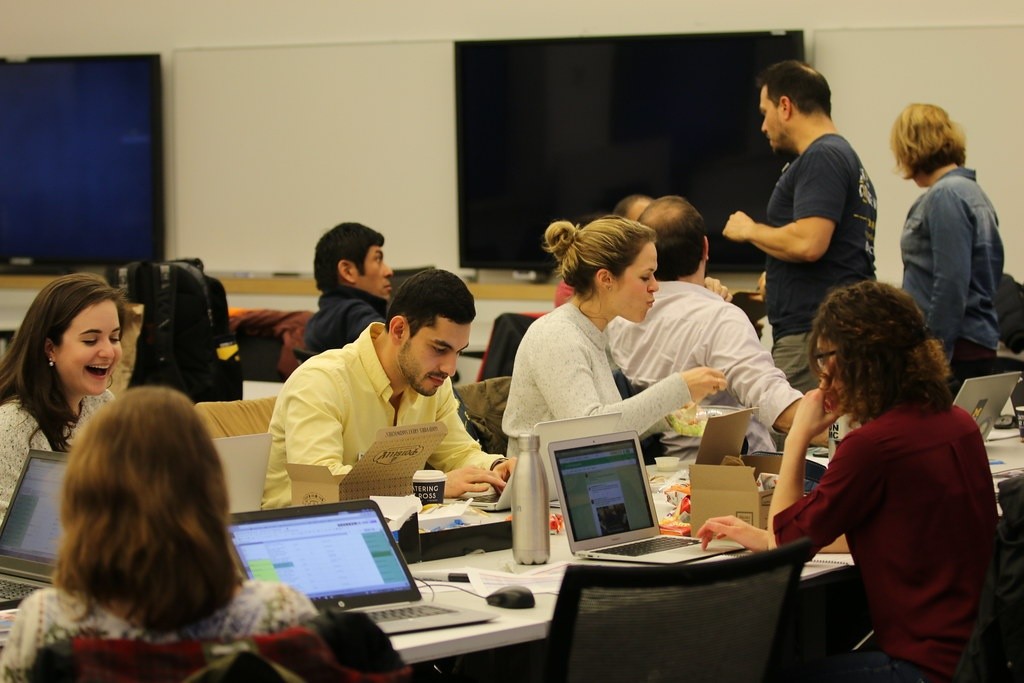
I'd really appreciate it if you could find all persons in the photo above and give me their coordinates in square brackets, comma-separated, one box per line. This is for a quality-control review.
[552, 196, 656, 310]
[720, 61, 877, 451]
[500, 212, 726, 460]
[301, 221, 394, 352]
[0, 273, 130, 527]
[889, 103, 1003, 392]
[262, 267, 518, 512]
[601, 196, 830, 454]
[0, 386, 322, 683]
[695, 281, 996, 683]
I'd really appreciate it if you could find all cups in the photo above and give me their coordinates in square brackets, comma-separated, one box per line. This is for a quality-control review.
[412, 469, 447, 505]
[1016, 406, 1024, 442]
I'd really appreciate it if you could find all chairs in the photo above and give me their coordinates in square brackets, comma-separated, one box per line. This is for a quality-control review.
[193, 395, 279, 440]
[475, 310, 552, 383]
[605, 349, 665, 466]
[529, 536, 815, 683]
[452, 376, 512, 457]
[228, 306, 316, 384]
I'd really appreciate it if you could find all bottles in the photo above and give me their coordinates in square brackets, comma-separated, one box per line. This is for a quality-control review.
[511, 432, 550, 564]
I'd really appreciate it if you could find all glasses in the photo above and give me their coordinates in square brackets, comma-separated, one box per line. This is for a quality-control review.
[815, 349, 840, 372]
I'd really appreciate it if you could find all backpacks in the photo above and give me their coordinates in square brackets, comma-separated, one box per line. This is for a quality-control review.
[125, 260, 243, 408]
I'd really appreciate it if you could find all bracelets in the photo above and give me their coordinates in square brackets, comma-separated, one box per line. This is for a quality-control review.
[490, 457, 509, 471]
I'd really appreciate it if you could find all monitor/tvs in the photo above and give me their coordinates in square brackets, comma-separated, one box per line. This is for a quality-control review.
[0, 52, 162, 275]
[454, 29, 805, 273]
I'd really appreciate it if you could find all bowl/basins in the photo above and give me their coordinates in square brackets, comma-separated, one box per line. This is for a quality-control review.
[655, 456, 680, 472]
[664, 406, 743, 438]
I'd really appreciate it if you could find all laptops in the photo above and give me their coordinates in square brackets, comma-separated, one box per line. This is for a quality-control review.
[229, 498, 499, 633]
[443, 412, 622, 510]
[0, 449, 69, 610]
[952, 371, 1021, 443]
[547, 430, 747, 564]
[212, 432, 273, 513]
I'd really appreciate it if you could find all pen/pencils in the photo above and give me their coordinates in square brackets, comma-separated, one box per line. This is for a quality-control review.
[273, 272, 300, 277]
[812, 453, 829, 458]
[989, 460, 1004, 464]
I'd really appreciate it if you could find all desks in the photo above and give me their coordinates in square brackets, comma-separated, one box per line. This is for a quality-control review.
[389, 535, 861, 664]
[443, 496, 512, 526]
[814, 435, 1024, 576]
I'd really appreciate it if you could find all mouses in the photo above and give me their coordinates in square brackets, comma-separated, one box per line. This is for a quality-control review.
[486, 585, 535, 608]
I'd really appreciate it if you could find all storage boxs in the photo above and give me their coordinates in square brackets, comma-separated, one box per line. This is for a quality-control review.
[287, 420, 448, 507]
[688, 405, 785, 538]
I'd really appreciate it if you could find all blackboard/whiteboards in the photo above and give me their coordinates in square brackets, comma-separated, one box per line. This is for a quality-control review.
[170, 39, 460, 294]
[811, 22, 1024, 291]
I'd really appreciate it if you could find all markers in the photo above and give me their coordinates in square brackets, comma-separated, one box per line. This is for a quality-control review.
[411, 571, 469, 583]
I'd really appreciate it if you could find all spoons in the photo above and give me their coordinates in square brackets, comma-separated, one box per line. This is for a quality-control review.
[680, 393, 710, 424]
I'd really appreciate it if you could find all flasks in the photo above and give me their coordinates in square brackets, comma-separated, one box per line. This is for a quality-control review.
[215, 341, 239, 361]
